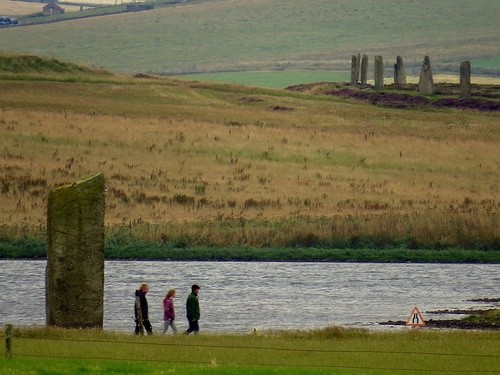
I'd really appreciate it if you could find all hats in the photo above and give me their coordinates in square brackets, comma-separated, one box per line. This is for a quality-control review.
[192, 285, 200, 290]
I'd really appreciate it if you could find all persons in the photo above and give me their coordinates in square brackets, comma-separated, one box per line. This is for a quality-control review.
[182, 284, 201, 336]
[132, 283, 155, 335]
[161, 289, 179, 337]
